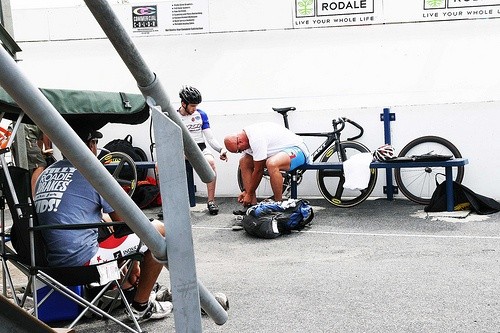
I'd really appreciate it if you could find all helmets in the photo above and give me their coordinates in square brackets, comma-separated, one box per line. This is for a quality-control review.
[373, 144, 395, 162]
[179, 87, 201, 104]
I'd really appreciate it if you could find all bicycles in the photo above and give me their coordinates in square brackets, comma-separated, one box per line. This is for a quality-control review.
[237, 106, 378, 208]
[97, 152, 139, 199]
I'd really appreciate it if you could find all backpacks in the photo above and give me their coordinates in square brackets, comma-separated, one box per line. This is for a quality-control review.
[98, 135, 133, 159]
[243, 200, 314, 239]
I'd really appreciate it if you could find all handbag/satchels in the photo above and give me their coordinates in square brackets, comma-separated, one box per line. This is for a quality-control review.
[132, 183, 162, 209]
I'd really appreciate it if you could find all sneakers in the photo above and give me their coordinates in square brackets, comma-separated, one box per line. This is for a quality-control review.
[150, 282, 168, 301]
[208, 201, 219, 214]
[125, 298, 172, 325]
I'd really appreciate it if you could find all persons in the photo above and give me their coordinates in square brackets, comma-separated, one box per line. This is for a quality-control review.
[224, 122, 309, 215]
[174, 86, 228, 214]
[12, 121, 54, 198]
[33, 122, 173, 322]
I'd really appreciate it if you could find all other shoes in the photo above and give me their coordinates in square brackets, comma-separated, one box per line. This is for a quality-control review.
[233, 209, 247, 214]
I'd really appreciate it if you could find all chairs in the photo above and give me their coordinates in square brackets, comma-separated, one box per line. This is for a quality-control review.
[0, 165, 144, 333]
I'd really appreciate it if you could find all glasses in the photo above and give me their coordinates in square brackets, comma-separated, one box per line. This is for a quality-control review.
[235, 137, 241, 153]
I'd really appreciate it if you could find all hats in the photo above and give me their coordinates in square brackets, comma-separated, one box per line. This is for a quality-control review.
[72, 125, 103, 144]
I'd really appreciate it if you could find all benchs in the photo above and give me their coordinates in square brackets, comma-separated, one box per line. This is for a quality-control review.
[107, 161, 196, 207]
[290, 157, 470, 217]
